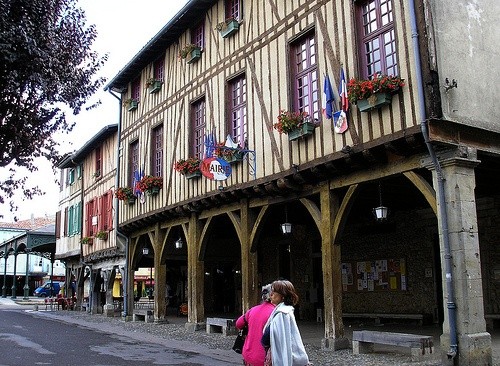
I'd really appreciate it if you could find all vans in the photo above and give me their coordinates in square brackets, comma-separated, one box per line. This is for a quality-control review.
[33, 282, 76, 299]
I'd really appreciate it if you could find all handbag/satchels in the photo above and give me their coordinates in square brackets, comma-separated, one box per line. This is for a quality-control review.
[232, 315, 247, 354]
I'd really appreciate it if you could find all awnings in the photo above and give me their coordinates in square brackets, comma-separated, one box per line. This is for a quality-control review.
[103, 0, 219, 102]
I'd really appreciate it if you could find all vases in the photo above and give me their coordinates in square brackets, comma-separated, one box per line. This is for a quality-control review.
[126, 88, 392, 206]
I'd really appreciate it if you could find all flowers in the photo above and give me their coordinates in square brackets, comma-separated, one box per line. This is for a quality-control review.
[345, 74, 405, 103]
[274, 110, 307, 134]
[174, 157, 201, 175]
[215, 142, 241, 161]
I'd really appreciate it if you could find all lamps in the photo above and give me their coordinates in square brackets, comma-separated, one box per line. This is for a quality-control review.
[280, 206, 293, 236]
[372, 181, 392, 223]
[142, 234, 184, 256]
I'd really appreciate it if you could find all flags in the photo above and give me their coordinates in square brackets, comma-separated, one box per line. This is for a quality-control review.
[338, 69, 349, 112]
[322, 75, 335, 119]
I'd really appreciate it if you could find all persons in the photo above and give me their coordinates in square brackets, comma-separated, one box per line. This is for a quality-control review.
[236, 284, 276, 366]
[260, 280, 309, 366]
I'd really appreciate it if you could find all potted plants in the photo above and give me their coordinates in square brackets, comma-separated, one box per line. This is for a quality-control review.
[146, 78, 162, 93]
[178, 44, 202, 65]
[215, 17, 239, 38]
[136, 175, 163, 192]
[123, 99, 138, 112]
[115, 187, 135, 200]
[82, 230, 108, 245]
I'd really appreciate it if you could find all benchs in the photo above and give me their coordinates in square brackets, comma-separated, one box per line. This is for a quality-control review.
[342, 313, 424, 325]
[132, 309, 154, 323]
[207, 317, 237, 335]
[352, 330, 434, 362]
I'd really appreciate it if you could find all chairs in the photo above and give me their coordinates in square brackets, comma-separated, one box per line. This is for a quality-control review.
[45, 294, 89, 312]
